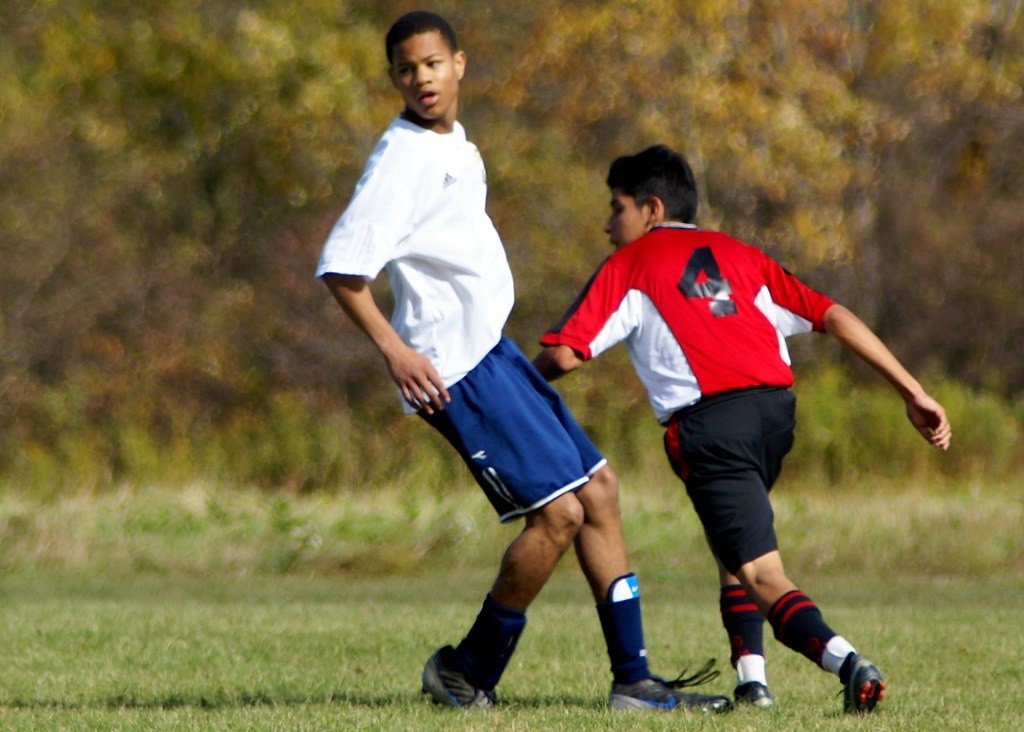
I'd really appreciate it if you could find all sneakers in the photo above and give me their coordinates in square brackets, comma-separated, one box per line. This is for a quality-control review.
[839, 656, 886, 713]
[422, 645, 497, 710]
[610, 658, 734, 715]
[734, 681, 773, 707]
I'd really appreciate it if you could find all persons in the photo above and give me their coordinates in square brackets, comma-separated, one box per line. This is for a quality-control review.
[531, 144, 952, 714]
[315, 9, 734, 715]
